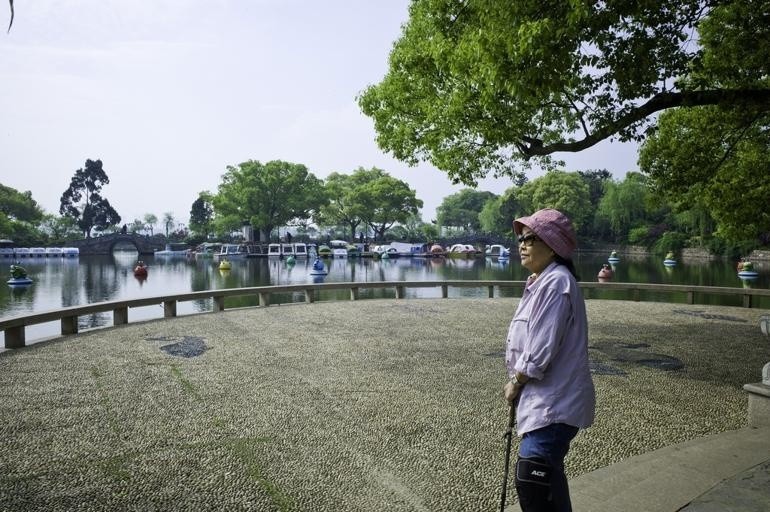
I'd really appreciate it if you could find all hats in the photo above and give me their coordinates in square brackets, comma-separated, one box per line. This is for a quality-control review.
[512, 208, 578, 261]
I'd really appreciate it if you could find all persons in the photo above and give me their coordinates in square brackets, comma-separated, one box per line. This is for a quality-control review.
[501, 206, 598, 511]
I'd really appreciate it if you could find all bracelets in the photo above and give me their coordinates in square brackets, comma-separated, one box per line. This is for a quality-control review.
[509, 374, 523, 388]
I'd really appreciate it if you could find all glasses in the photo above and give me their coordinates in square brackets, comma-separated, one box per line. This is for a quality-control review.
[515, 232, 540, 246]
